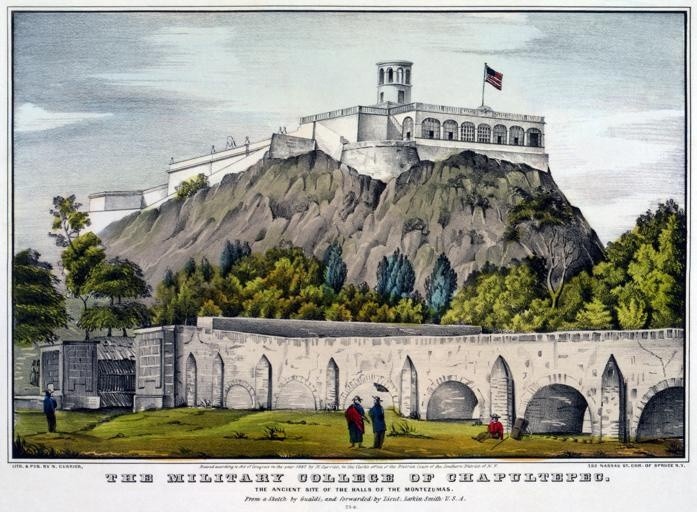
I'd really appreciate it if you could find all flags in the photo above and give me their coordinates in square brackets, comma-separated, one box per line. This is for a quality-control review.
[484, 64, 502, 91]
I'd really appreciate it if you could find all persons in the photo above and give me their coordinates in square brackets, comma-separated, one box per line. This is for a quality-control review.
[471, 413, 502, 443]
[343, 395, 371, 448]
[367, 395, 386, 449]
[42, 390, 57, 432]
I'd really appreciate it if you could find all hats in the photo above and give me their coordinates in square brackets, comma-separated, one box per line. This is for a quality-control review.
[490, 414, 500, 419]
[372, 396, 382, 402]
[352, 397, 362, 401]
